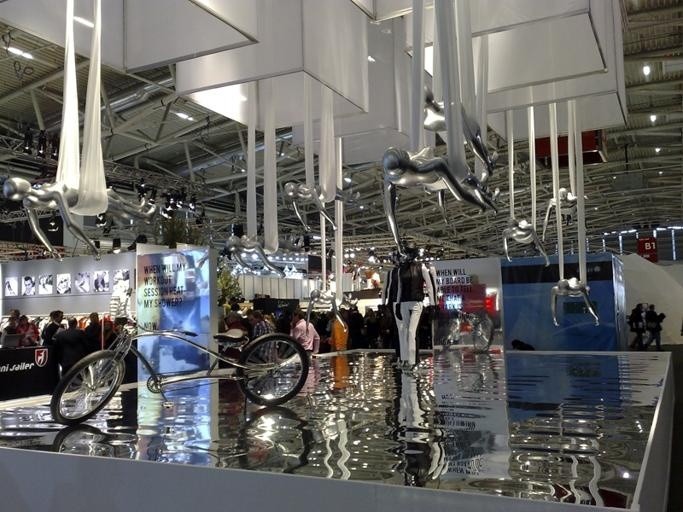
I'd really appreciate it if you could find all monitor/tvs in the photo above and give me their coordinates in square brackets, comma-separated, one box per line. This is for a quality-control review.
[443, 293, 463, 310]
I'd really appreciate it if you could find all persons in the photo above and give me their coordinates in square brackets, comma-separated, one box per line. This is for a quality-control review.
[225, 234, 305, 279]
[549, 276, 600, 328]
[397, 371, 450, 487]
[94, 272, 107, 292]
[629, 303, 645, 350]
[644, 304, 664, 350]
[57, 274, 71, 294]
[22, 276, 35, 296]
[423, 86, 499, 175]
[39, 275, 53, 294]
[283, 181, 361, 232]
[376, 146, 503, 257]
[681, 320, 683, 336]
[379, 238, 438, 370]
[3, 176, 157, 262]
[542, 188, 589, 240]
[501, 217, 551, 269]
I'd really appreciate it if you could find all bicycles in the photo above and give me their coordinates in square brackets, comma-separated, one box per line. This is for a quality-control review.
[449, 306, 494, 352]
[51, 287, 309, 425]
[53, 405, 311, 474]
